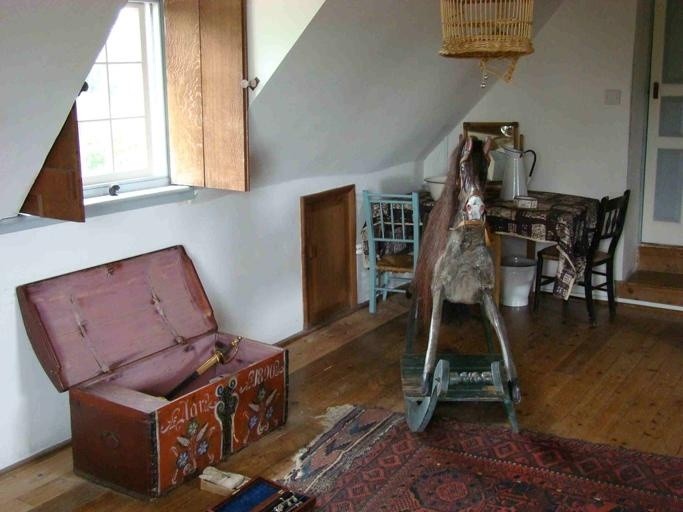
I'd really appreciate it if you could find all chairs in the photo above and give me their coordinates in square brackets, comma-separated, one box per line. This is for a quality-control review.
[363, 191, 420, 314]
[533, 189, 630, 328]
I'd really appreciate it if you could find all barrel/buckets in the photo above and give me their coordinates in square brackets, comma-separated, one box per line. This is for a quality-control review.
[499, 257, 537, 307]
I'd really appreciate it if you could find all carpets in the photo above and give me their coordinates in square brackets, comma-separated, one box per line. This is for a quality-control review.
[275, 405, 683, 512]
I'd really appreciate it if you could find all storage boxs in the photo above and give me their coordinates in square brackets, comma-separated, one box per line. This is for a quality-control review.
[16, 246, 291, 504]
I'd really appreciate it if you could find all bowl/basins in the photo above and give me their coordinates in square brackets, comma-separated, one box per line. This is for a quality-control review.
[423, 176, 447, 202]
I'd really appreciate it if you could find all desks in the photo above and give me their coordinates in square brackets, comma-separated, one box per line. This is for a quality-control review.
[362, 190, 600, 303]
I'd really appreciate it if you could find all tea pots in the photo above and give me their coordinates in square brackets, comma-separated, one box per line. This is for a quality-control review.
[501, 145, 536, 201]
